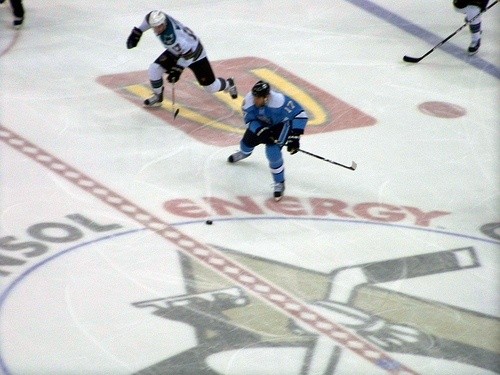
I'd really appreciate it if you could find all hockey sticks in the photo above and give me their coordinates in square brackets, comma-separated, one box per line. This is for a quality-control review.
[402, 0, 499, 64]
[171, 77, 180, 120]
[275, 140, 358, 171]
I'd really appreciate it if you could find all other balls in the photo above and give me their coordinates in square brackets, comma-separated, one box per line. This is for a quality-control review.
[206, 221, 212, 224]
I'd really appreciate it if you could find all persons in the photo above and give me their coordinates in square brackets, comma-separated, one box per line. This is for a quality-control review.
[227, 81, 307, 198]
[126, 10, 237, 105]
[452, 0, 489, 56]
[0, 0, 24, 26]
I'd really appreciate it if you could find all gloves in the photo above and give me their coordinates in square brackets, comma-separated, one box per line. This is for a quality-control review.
[287, 130, 300, 155]
[167, 64, 185, 83]
[126, 27, 142, 49]
[255, 125, 277, 146]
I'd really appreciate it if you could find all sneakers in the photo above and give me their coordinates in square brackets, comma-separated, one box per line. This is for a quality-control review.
[227, 78, 237, 99]
[468, 38, 481, 56]
[144, 86, 165, 108]
[13, 17, 24, 30]
[273, 182, 284, 202]
[227, 151, 252, 163]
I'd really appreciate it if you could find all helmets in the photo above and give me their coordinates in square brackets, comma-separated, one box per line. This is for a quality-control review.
[251, 80, 270, 97]
[149, 11, 166, 27]
